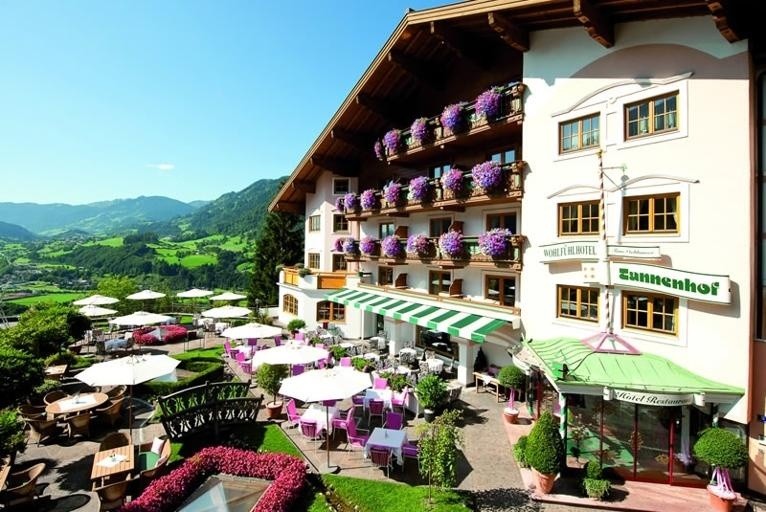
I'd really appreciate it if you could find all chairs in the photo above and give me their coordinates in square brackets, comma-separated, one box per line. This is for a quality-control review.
[0, 384, 171, 510]
[225, 331, 462, 473]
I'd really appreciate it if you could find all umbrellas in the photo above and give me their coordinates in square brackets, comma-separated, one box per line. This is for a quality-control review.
[67, 287, 252, 355]
[217, 320, 283, 382]
[275, 365, 371, 468]
[77, 352, 182, 437]
[255, 341, 332, 377]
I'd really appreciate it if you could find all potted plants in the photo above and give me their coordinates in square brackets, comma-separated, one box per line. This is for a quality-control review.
[513, 437, 529, 468]
[497, 364, 525, 423]
[526, 411, 564, 493]
[579, 459, 609, 501]
[693, 428, 749, 512]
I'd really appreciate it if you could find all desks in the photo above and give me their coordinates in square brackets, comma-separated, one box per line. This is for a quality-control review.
[472, 369, 512, 403]
